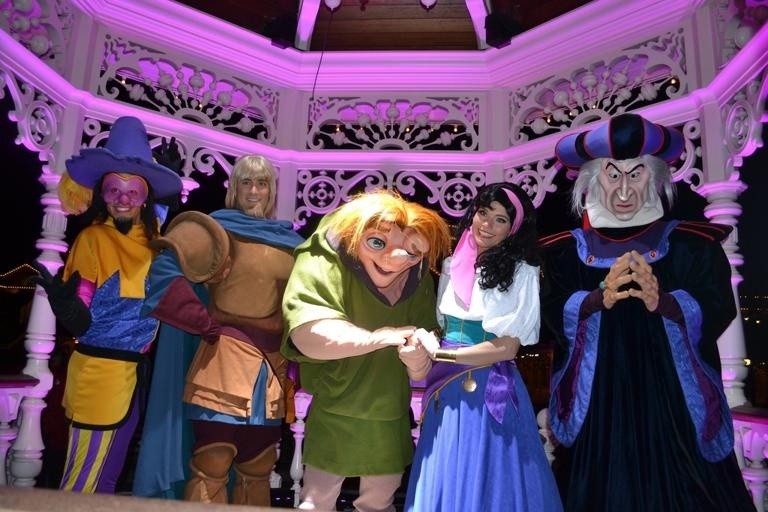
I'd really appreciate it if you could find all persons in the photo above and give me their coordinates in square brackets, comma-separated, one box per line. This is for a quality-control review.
[533, 115, 756, 512]
[145, 155, 307, 509]
[412, 182, 565, 512]
[281, 188, 451, 512]
[27, 116, 183, 494]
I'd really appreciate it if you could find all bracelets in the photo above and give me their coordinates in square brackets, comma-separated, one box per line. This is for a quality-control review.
[435, 345, 456, 363]
